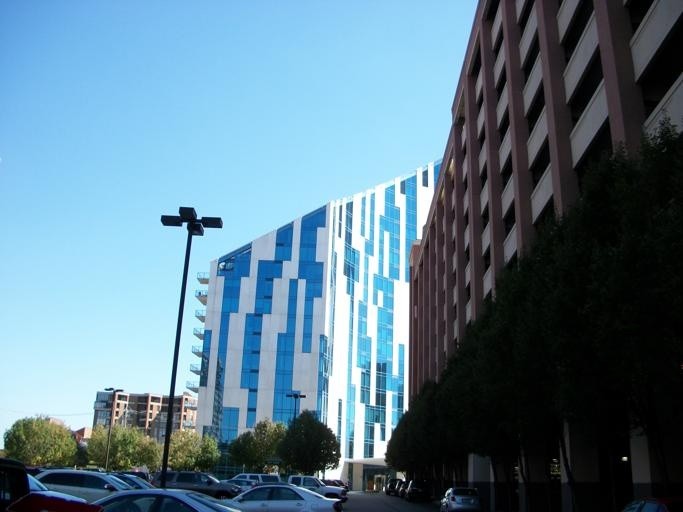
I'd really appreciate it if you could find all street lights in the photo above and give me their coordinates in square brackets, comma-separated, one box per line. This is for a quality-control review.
[159, 207, 222, 488]
[287, 395, 306, 435]
[104, 388, 122, 471]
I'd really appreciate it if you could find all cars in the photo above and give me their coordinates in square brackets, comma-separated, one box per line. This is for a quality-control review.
[622, 496, 683, 512]
[385, 478, 479, 511]
